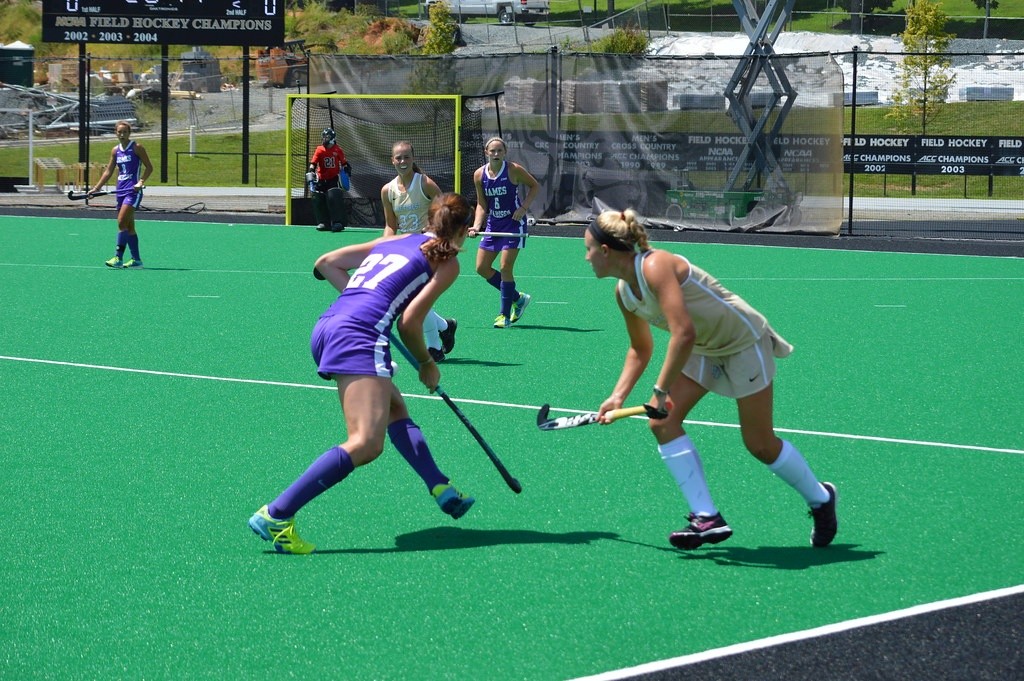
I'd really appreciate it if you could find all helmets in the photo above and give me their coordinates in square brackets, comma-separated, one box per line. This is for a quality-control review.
[320, 127, 336, 146]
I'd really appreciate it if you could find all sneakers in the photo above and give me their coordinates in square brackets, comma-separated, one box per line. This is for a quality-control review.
[438, 318, 458, 354]
[248, 504, 317, 555]
[807, 482, 838, 547]
[670, 510, 732, 550]
[431, 480, 476, 519]
[119, 258, 144, 269]
[105, 255, 123, 268]
[494, 314, 512, 328]
[509, 292, 531, 323]
[427, 347, 446, 363]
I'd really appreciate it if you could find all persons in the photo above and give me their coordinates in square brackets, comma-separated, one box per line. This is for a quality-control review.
[467, 138, 540, 329]
[244, 192, 472, 554]
[306, 128, 351, 232]
[583, 207, 837, 550]
[380, 141, 458, 364]
[87, 122, 154, 270]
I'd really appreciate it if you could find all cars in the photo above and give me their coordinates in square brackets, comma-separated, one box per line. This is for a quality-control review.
[424, 0, 550, 26]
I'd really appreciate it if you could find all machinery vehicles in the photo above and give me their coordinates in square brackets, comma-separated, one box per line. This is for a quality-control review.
[254, 38, 340, 86]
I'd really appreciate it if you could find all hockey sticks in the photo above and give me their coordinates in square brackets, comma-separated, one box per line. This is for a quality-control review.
[388, 332, 523, 493]
[67, 185, 147, 200]
[462, 232, 529, 238]
[536, 403, 674, 430]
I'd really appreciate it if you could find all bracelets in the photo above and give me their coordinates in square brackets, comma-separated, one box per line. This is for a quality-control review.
[140, 178, 144, 182]
[652, 384, 670, 396]
[416, 357, 435, 366]
[520, 206, 527, 211]
[94, 185, 99, 192]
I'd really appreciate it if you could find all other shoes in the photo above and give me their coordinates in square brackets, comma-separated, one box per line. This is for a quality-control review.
[317, 222, 328, 231]
[331, 222, 344, 232]
